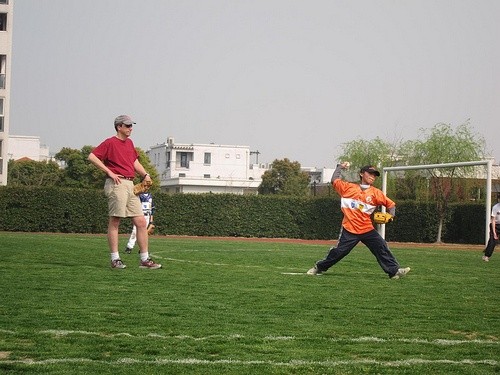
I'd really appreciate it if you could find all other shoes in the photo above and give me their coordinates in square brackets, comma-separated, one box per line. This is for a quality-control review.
[125, 248, 131, 254]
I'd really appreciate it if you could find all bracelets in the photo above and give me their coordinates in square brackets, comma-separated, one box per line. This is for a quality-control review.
[143, 173, 149, 178]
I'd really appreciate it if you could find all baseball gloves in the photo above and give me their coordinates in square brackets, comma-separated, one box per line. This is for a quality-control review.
[147, 223, 155, 235]
[372, 211, 393, 224]
[134, 181, 151, 196]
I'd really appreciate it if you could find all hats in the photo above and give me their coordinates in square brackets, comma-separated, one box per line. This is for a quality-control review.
[360, 164, 380, 176]
[114, 114, 136, 125]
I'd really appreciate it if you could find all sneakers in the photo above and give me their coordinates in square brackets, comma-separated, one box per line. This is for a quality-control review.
[139, 257, 162, 269]
[391, 267, 410, 281]
[111, 258, 126, 269]
[307, 266, 320, 275]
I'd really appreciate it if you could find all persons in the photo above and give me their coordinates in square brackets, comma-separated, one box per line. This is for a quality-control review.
[306, 159, 411, 281]
[87, 115, 162, 270]
[123, 177, 156, 254]
[481, 194, 500, 262]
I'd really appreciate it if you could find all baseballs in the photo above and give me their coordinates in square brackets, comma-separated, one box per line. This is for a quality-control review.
[344, 161, 350, 167]
